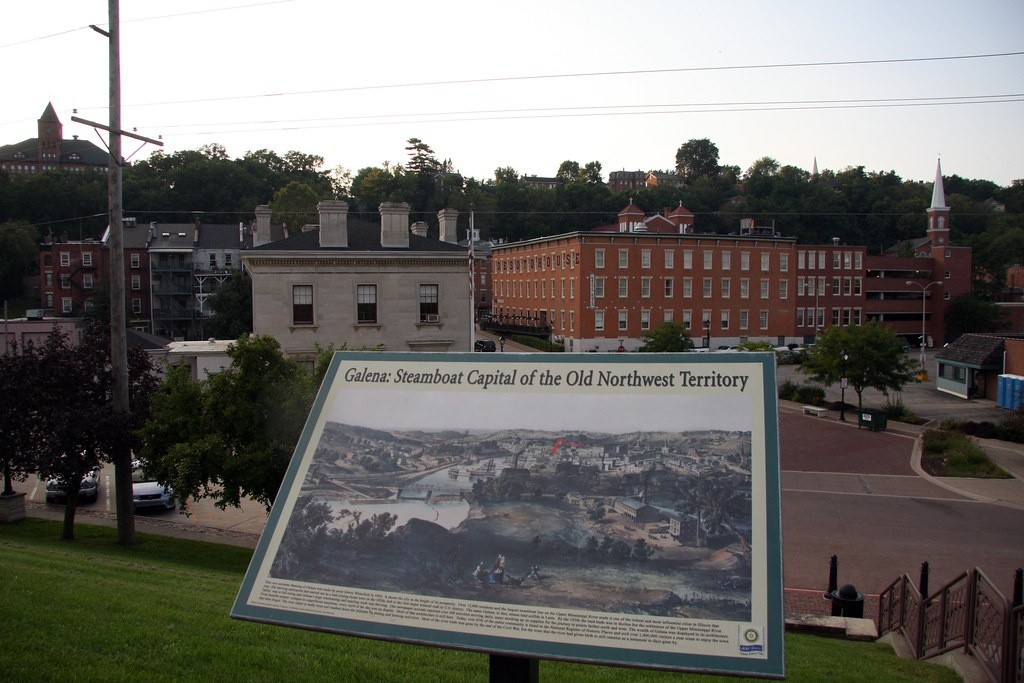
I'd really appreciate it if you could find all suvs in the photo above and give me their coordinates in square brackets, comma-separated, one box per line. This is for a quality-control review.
[475, 339, 496, 352]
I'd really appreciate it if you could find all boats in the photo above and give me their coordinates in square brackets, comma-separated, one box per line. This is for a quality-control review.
[448, 466, 459, 476]
[348, 493, 400, 504]
[430, 492, 462, 501]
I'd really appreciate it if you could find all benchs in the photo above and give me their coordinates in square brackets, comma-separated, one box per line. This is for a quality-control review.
[802, 405, 827, 418]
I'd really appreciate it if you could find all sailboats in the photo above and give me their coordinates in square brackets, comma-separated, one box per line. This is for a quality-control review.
[470, 458, 497, 477]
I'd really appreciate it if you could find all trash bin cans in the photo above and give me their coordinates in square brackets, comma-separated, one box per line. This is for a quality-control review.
[856, 407, 888, 431]
[830, 582, 865, 619]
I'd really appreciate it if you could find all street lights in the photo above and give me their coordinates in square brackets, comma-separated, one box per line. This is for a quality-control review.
[707, 319, 710, 347]
[498, 335, 505, 352]
[839, 348, 850, 422]
[550, 320, 553, 352]
[906, 279, 943, 370]
[804, 283, 830, 334]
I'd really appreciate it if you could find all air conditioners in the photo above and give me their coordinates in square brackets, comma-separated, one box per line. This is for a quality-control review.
[427, 315, 439, 322]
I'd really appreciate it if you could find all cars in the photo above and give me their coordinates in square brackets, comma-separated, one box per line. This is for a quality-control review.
[788, 343, 799, 349]
[728, 344, 748, 350]
[791, 348, 807, 355]
[773, 346, 790, 355]
[130, 458, 175, 514]
[45, 450, 101, 504]
[716, 345, 730, 350]
[687, 348, 709, 352]
[807, 344, 816, 348]
